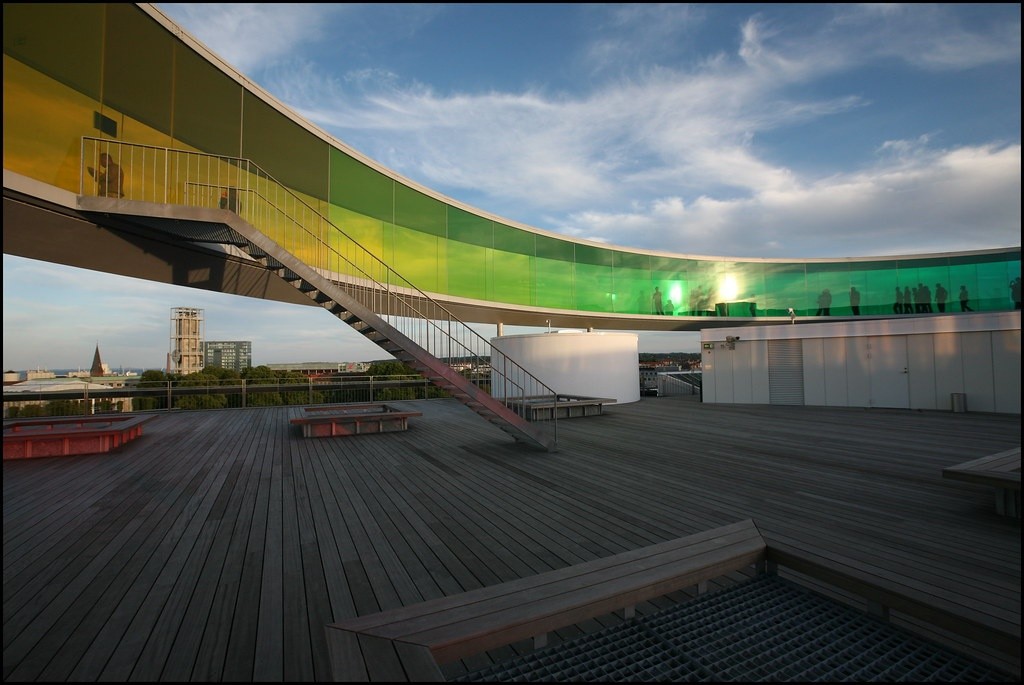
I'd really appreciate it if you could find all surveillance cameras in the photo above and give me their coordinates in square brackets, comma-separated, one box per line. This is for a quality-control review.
[736, 336, 740, 340]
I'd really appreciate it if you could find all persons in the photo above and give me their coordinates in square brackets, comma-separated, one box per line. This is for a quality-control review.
[892, 282, 974, 314]
[850, 286, 860, 315]
[749, 294, 756, 317]
[689, 285, 727, 317]
[815, 289, 832, 316]
[95, 153, 125, 199]
[1010, 277, 1022, 310]
[653, 286, 675, 315]
[220, 185, 242, 216]
